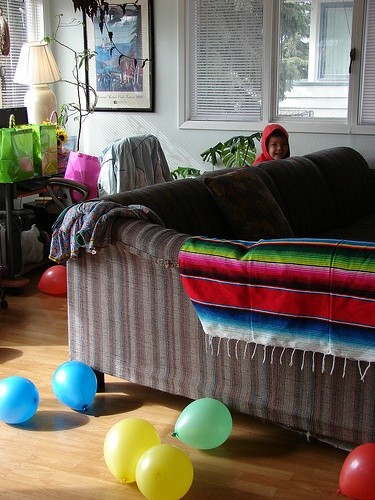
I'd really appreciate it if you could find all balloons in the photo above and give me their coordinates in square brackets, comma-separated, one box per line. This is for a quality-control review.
[0, 376, 39, 424]
[53, 361, 99, 411]
[36, 265, 68, 296]
[136, 445, 194, 500]
[337, 442, 375, 500]
[172, 398, 232, 450]
[104, 419, 162, 485]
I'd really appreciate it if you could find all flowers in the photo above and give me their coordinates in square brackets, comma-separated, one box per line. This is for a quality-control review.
[42, 121, 67, 149]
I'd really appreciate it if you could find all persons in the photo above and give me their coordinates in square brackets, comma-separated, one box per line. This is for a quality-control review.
[252, 124, 290, 167]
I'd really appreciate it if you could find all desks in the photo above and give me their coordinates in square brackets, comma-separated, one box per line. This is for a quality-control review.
[0, 172, 65, 279]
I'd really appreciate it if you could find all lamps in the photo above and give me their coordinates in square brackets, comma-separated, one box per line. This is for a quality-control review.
[14, 42, 62, 125]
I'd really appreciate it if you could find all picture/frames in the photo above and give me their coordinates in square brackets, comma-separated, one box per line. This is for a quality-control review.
[83, 0, 155, 113]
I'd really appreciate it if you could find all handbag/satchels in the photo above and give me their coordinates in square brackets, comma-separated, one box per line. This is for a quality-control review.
[0, 114, 34, 183]
[64, 152, 100, 203]
[33, 111, 58, 177]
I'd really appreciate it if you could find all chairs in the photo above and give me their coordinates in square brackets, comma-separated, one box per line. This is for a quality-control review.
[37, 134, 176, 266]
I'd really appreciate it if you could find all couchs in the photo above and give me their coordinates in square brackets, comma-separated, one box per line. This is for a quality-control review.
[68, 147, 375, 455]
